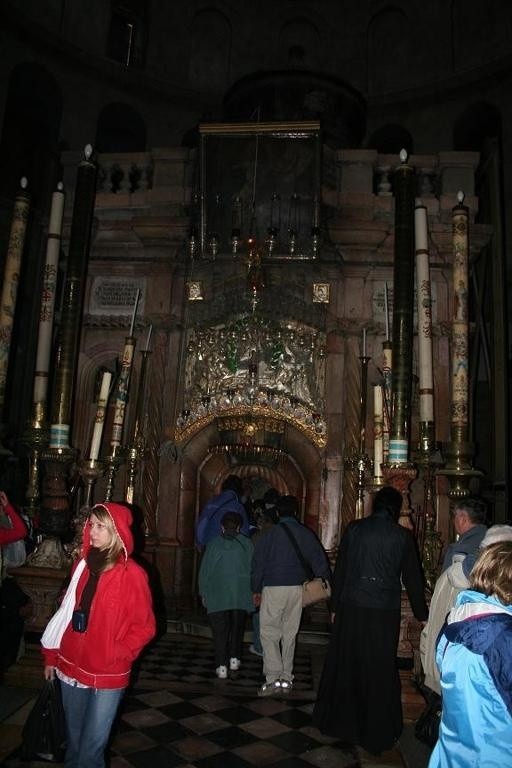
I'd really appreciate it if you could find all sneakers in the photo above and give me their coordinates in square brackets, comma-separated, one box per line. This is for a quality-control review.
[215, 665, 227, 679]
[229, 657, 240, 671]
[257, 680, 293, 696]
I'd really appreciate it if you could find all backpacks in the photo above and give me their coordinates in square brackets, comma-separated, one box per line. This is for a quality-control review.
[19, 675, 68, 764]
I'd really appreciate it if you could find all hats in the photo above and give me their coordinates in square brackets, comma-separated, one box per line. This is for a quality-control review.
[479, 523, 511, 548]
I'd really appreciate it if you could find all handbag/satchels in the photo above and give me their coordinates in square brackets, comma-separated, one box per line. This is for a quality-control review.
[302, 577, 333, 610]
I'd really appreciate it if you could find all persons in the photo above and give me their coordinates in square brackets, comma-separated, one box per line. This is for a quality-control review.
[426, 541, 511, 768]
[249, 486, 286, 534]
[443, 499, 490, 573]
[309, 485, 429, 758]
[196, 475, 250, 553]
[0, 491, 29, 545]
[197, 511, 257, 679]
[421, 523, 511, 704]
[250, 494, 333, 698]
[38, 501, 159, 767]
